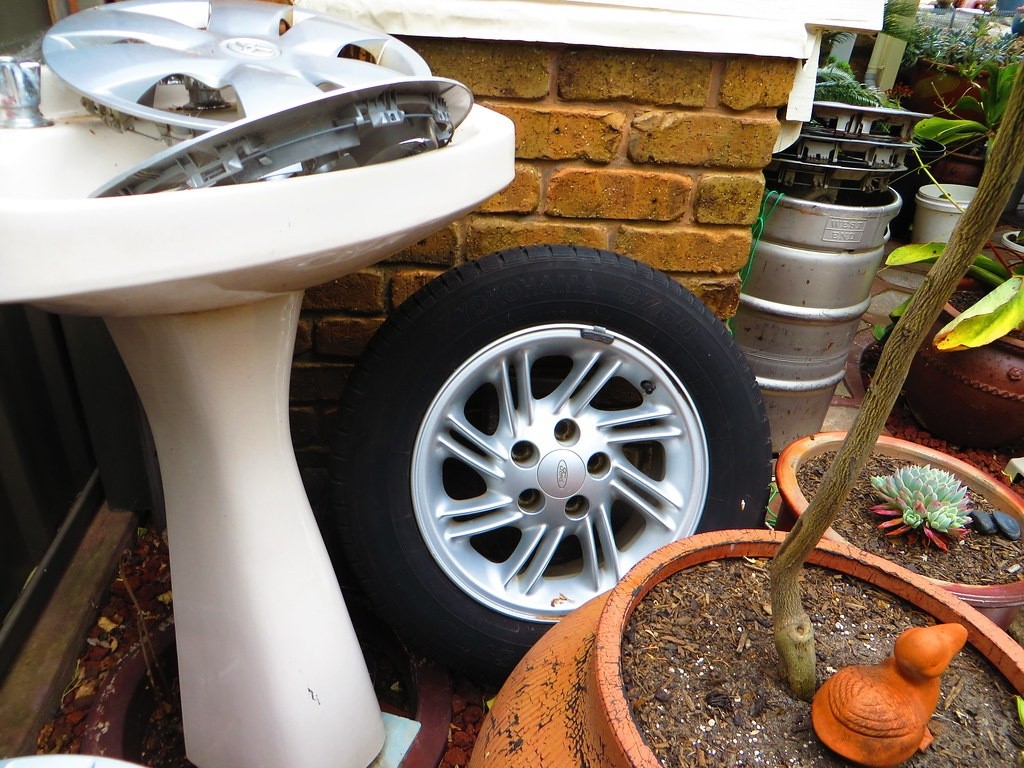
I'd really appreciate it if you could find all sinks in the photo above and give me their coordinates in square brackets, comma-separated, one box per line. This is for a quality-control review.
[0, 22, 516, 319]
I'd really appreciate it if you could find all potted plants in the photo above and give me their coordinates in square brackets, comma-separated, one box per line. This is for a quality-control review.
[460, 0, 1023, 768]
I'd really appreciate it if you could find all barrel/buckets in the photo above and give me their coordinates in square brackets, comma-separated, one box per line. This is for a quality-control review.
[915, 184, 979, 245]
[907, 137, 945, 179]
[733, 181, 904, 456]
